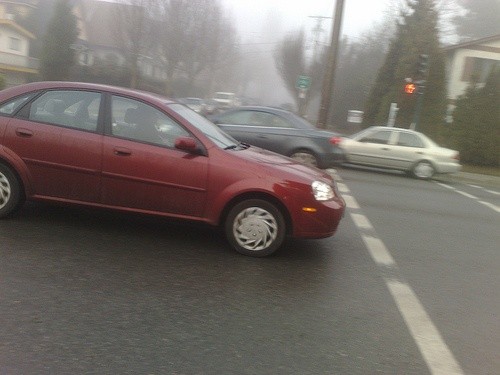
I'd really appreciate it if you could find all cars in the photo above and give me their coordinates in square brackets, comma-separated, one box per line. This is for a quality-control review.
[207, 105, 343, 169]
[181, 94, 206, 117]
[0, 82, 346, 257]
[341, 124, 462, 179]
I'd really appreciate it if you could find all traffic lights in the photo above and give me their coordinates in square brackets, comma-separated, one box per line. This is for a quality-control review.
[402, 79, 415, 96]
[416, 45, 432, 96]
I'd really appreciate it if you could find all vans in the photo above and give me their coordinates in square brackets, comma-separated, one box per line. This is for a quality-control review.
[214, 92, 236, 110]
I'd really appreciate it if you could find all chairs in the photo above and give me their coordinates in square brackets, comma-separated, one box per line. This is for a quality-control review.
[23, 98, 85, 129]
[123, 107, 146, 140]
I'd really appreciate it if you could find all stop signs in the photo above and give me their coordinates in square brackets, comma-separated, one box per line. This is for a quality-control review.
[295, 71, 313, 93]
